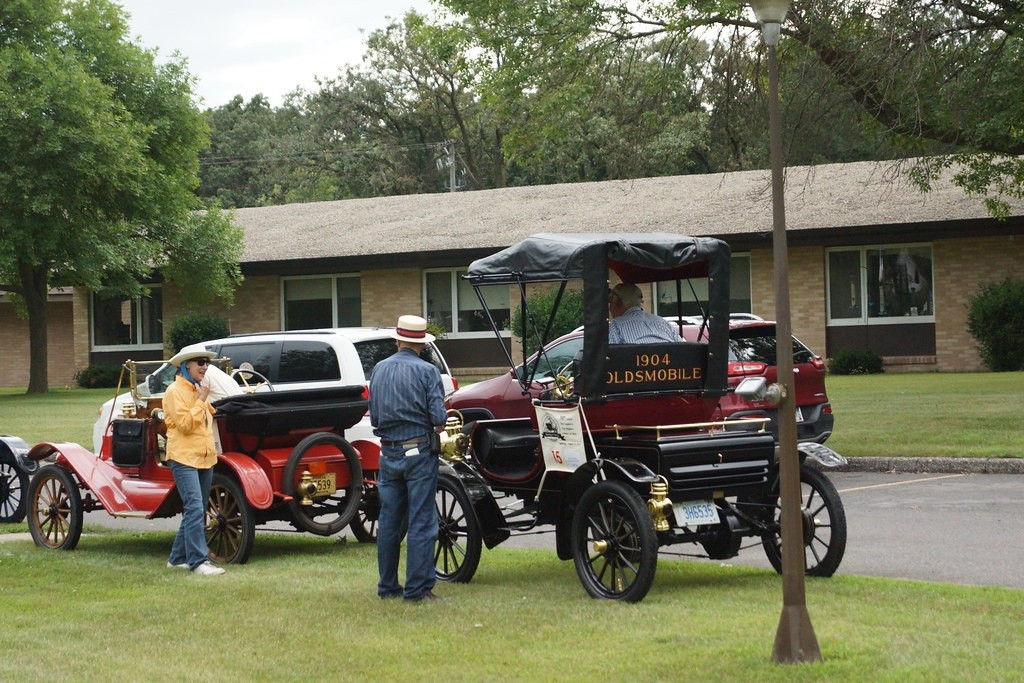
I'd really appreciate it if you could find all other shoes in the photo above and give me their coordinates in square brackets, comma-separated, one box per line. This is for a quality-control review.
[420, 591, 445, 601]
[190, 561, 224, 575]
[166, 562, 188, 568]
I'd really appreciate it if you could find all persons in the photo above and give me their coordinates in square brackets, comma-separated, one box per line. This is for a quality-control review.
[608, 283, 687, 345]
[162, 344, 227, 576]
[368, 315, 448, 604]
[174, 363, 244, 403]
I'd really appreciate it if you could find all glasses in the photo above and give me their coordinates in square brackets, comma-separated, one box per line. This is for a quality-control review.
[188, 358, 210, 365]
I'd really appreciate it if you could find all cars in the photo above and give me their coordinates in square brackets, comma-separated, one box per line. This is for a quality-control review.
[16, 356, 412, 570]
[401, 228, 851, 606]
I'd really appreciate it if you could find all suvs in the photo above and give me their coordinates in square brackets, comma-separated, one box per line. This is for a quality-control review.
[442, 306, 833, 512]
[88, 323, 459, 471]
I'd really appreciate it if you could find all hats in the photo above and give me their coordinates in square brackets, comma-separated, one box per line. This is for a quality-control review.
[388, 314, 435, 342]
[169, 343, 216, 366]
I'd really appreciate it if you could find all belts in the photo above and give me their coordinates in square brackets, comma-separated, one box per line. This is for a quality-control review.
[380, 435, 428, 444]
